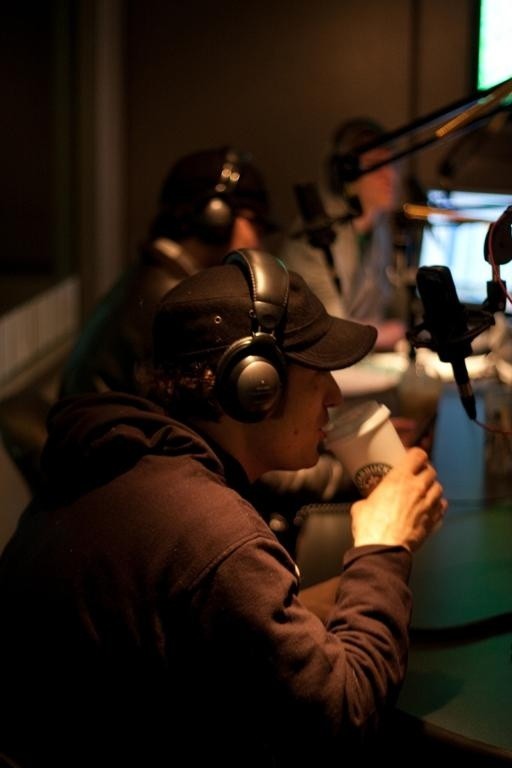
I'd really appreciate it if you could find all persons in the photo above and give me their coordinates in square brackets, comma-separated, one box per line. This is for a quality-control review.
[281, 119, 425, 351]
[43, 147, 432, 506]
[0, 249, 448, 767]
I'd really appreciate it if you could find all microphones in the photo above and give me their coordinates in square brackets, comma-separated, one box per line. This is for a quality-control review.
[291, 176, 341, 293]
[415, 265, 478, 423]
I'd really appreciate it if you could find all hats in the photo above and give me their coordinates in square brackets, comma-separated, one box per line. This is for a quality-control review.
[157, 150, 280, 237]
[151, 262, 378, 370]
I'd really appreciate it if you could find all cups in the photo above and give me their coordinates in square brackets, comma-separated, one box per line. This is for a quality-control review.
[321, 400, 442, 537]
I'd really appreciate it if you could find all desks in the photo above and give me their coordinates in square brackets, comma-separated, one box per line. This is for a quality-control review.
[294, 330, 512, 761]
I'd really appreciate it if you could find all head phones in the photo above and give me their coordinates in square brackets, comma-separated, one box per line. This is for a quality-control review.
[216, 247, 292, 424]
[191, 146, 244, 249]
